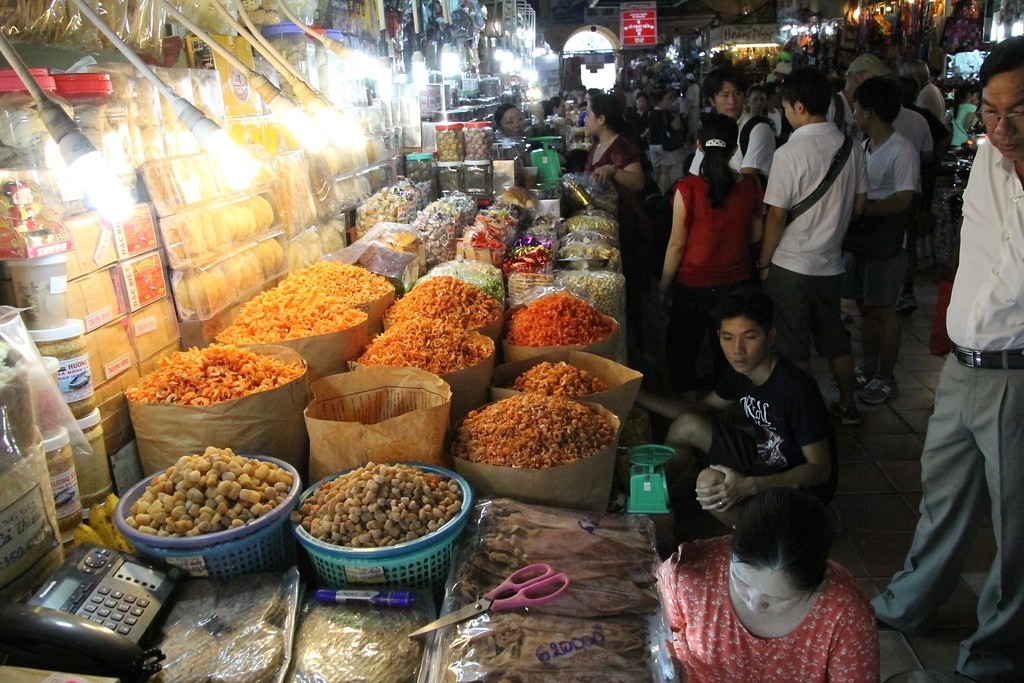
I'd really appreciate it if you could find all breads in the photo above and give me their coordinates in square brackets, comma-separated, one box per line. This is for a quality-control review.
[504, 186, 535, 207]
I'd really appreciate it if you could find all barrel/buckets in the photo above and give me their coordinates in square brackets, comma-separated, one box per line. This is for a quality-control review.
[6, 253, 67, 329]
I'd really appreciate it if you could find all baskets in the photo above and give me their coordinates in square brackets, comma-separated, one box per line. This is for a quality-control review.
[292, 461, 473, 594]
[115, 453, 301, 579]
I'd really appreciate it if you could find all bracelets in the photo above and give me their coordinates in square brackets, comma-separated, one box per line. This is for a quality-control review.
[612, 164, 617, 175]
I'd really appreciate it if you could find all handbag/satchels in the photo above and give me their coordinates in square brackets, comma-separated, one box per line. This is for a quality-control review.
[641, 177, 673, 246]
[940, 5, 983, 55]
[661, 110, 683, 150]
[841, 39, 856, 51]
[885, 44, 899, 58]
[841, 215, 907, 260]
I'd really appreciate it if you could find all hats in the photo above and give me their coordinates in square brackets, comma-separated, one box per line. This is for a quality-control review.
[685, 73, 694, 79]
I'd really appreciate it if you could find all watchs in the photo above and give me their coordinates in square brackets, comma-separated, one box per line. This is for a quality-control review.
[756, 261, 770, 271]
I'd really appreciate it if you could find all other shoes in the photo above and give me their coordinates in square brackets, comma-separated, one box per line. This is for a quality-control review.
[839, 405, 861, 425]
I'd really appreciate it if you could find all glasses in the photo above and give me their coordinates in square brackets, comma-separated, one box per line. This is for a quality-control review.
[976, 113, 1024, 127]
[843, 69, 868, 78]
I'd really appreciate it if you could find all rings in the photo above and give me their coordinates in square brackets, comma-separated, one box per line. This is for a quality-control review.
[719, 501, 724, 506]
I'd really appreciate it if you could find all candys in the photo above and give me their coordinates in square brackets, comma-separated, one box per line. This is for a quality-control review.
[410, 190, 560, 276]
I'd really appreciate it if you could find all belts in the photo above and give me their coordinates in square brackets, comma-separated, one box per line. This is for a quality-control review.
[950, 340, 1024, 370]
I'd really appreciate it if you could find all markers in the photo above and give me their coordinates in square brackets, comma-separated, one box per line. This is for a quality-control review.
[316, 589, 415, 606]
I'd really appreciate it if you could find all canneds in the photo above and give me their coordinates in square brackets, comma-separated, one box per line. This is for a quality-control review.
[20, 317, 113, 531]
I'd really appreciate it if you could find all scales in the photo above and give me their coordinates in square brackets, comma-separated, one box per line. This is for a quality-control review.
[528, 136, 562, 189]
[627, 443, 675, 513]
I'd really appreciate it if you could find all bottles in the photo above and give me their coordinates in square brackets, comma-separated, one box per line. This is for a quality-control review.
[405, 122, 494, 200]
[729, 47, 781, 67]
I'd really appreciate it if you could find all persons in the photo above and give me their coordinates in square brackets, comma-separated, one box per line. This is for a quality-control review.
[689, 64, 775, 179]
[613, 73, 701, 195]
[742, 71, 791, 148]
[657, 114, 765, 404]
[494, 103, 568, 164]
[870, 38, 1024, 683]
[583, 93, 652, 320]
[950, 84, 978, 146]
[826, 53, 947, 403]
[578, 88, 601, 127]
[634, 292, 844, 533]
[658, 488, 881, 683]
[755, 66, 869, 426]
[542, 97, 566, 117]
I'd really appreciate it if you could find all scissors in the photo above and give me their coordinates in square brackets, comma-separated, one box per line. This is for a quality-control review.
[409, 563, 569, 638]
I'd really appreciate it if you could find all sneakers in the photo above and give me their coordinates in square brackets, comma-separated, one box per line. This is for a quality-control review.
[895, 294, 917, 312]
[830, 367, 869, 387]
[858, 379, 899, 403]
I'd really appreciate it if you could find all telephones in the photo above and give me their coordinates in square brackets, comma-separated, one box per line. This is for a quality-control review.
[0, 540, 181, 675]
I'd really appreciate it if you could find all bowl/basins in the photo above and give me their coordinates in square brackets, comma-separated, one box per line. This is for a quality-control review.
[557, 258, 610, 270]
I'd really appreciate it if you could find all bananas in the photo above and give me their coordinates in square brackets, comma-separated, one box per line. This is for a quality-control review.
[74, 491, 141, 556]
